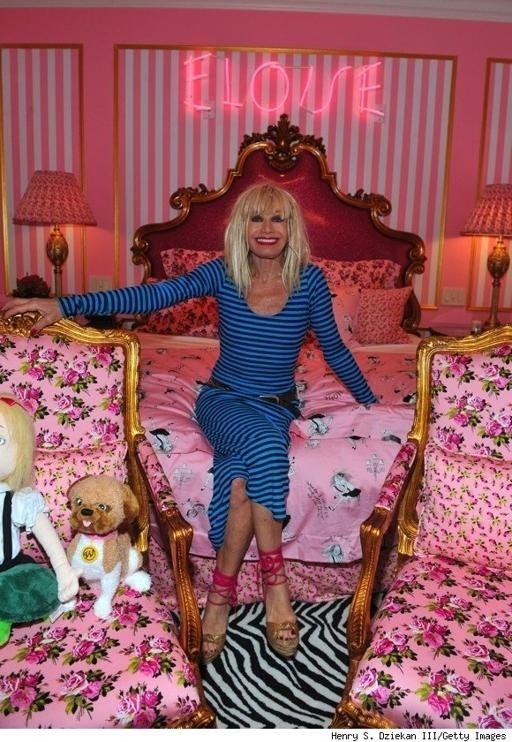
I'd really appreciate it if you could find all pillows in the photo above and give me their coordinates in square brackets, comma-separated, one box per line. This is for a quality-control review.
[414, 441, 511, 573]
[20, 441, 128, 564]
[142, 248, 415, 348]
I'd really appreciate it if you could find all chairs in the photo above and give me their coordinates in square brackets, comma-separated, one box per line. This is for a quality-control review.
[0, 314, 215, 728]
[328, 326, 512, 728]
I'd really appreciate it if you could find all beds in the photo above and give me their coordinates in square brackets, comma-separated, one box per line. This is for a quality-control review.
[131, 114, 427, 600]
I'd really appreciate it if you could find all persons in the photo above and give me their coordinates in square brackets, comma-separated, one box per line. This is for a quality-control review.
[1, 181, 380, 662]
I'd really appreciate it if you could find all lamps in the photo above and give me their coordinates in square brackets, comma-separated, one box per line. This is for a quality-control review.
[12, 170, 98, 298]
[459, 183, 511, 334]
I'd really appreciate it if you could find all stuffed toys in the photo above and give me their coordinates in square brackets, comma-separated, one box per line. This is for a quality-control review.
[65, 473, 152, 619]
[2, 395, 77, 648]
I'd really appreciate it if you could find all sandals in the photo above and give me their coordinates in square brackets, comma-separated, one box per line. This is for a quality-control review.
[259, 548, 298, 656]
[200, 568, 237, 666]
